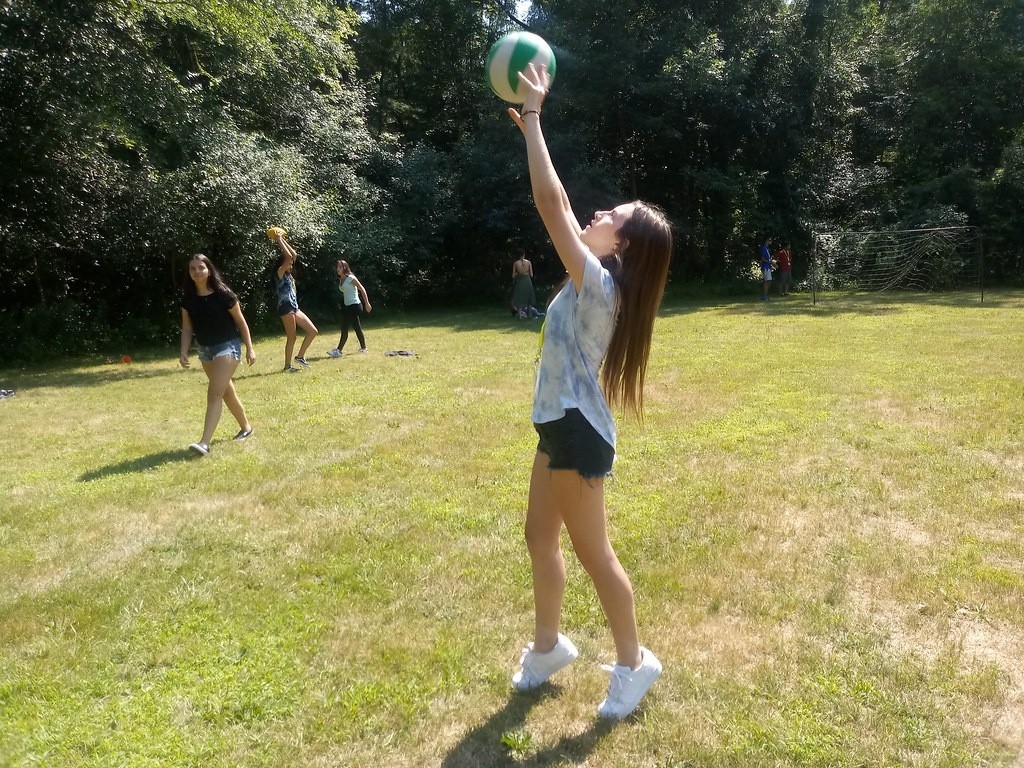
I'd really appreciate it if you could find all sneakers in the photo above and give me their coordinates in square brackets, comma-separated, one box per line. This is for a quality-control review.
[598, 646, 662, 720]
[511, 632, 578, 692]
[284, 365, 302, 373]
[294, 356, 311, 368]
[326, 349, 343, 357]
[357, 349, 368, 353]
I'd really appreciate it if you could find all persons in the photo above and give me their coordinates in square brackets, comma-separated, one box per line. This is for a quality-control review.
[180, 250, 256, 456]
[274, 234, 318, 372]
[774, 241, 793, 297]
[760, 236, 774, 302]
[513, 249, 534, 321]
[327, 261, 371, 357]
[506, 59, 675, 721]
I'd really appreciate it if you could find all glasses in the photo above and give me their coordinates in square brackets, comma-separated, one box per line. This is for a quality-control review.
[768, 239, 772, 241]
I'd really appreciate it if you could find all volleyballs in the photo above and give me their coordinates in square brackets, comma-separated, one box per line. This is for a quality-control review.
[484, 30, 557, 104]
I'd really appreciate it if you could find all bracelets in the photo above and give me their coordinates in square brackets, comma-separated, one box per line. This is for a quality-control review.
[520, 109, 541, 123]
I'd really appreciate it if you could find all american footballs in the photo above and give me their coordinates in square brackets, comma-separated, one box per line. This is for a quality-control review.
[266, 227, 288, 241]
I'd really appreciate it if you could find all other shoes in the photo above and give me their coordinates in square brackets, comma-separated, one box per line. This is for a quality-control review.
[232, 427, 254, 441]
[760, 297, 768, 301]
[189, 441, 210, 456]
